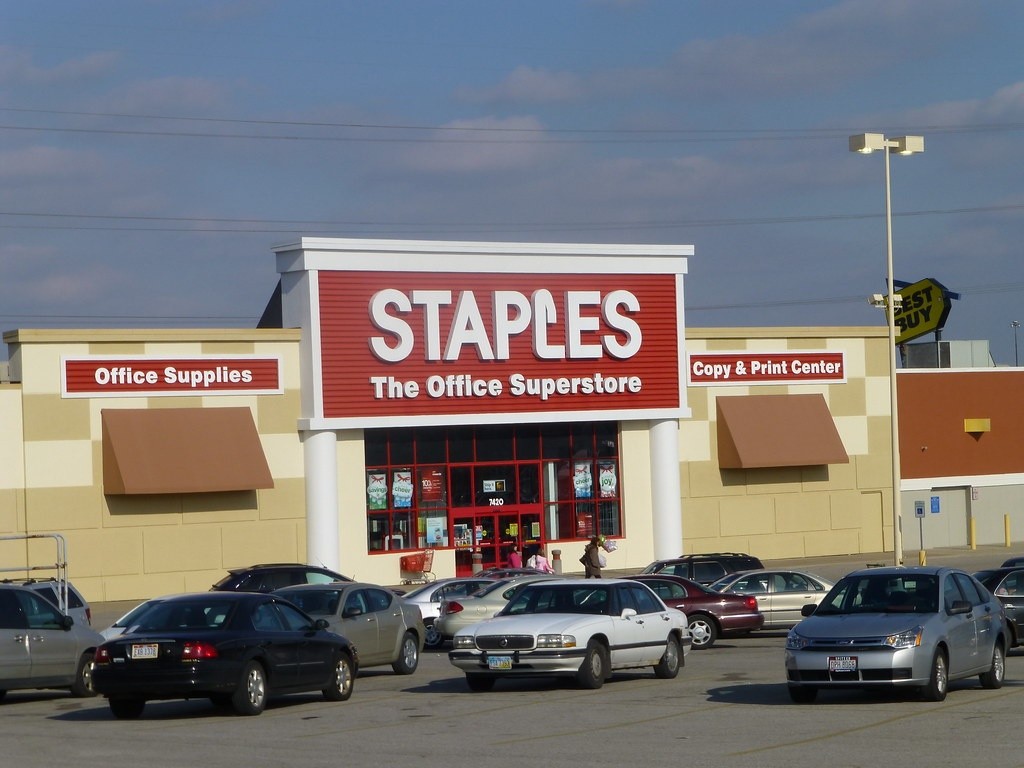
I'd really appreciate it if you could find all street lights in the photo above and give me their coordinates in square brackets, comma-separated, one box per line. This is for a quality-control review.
[849, 127, 925, 563]
[1010, 320, 1021, 366]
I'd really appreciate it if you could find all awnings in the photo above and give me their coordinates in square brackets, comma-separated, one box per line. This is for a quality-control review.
[102, 406, 274, 495]
[718, 393, 849, 468]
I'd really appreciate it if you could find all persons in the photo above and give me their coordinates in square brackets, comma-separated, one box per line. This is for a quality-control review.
[527, 543, 555, 574]
[584, 536, 602, 578]
[507, 544, 522, 569]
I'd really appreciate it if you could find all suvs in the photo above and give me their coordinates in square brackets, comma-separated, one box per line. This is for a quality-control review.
[639, 551, 765, 585]
[208, 561, 358, 593]
[3, 575, 92, 630]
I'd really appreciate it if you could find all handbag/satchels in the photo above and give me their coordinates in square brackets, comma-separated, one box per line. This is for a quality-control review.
[598, 554, 607, 567]
[579, 556, 586, 565]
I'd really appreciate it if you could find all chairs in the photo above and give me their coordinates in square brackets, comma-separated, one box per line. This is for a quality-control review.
[370, 597, 383, 610]
[187, 611, 208, 629]
[328, 599, 346, 614]
[747, 578, 766, 592]
[890, 588, 931, 611]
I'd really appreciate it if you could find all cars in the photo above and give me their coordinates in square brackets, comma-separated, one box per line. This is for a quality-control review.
[98, 593, 196, 641]
[1000, 556, 1024, 568]
[91, 589, 361, 718]
[270, 582, 426, 674]
[619, 572, 765, 650]
[972, 566, 1024, 654]
[784, 565, 1013, 703]
[0, 583, 106, 699]
[707, 569, 861, 631]
[435, 576, 565, 640]
[447, 577, 693, 689]
[400, 576, 500, 649]
